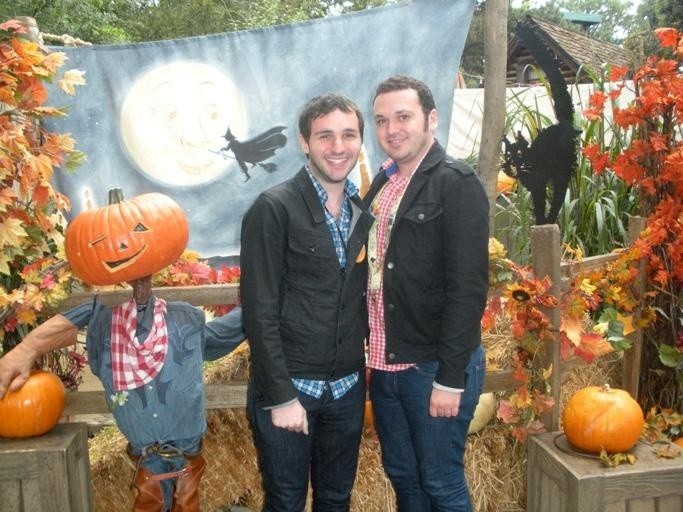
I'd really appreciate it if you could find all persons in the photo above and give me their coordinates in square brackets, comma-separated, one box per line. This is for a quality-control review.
[237, 94, 390, 511]
[217, 127, 255, 183]
[359, 77, 488, 512]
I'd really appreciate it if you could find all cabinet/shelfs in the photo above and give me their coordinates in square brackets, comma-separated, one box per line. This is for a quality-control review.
[524, 430, 682, 512]
[0, 423, 95, 512]
